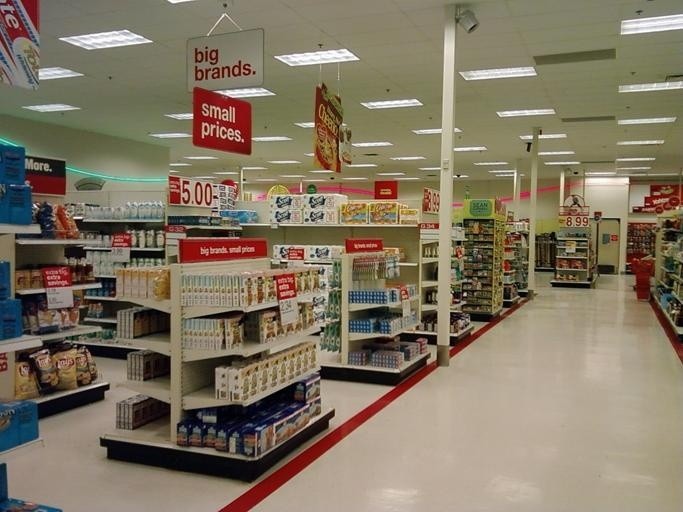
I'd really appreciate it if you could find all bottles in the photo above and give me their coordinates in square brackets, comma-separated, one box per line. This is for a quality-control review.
[126, 258, 166, 267]
[86, 279, 114, 296]
[68, 255, 95, 284]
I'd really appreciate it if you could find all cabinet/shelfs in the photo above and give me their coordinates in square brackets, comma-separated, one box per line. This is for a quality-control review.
[0, 197, 531, 512]
[534, 197, 597, 288]
[626, 213, 683, 341]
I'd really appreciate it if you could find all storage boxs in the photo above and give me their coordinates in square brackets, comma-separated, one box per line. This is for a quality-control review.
[0, 145, 32, 224]
